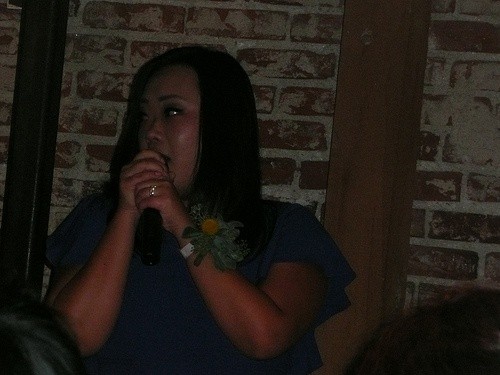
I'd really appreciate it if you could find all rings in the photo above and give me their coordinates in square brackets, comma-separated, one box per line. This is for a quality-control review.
[149, 185, 156, 196]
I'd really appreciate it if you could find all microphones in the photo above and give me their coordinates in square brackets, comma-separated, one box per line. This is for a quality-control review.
[139, 205, 163, 266]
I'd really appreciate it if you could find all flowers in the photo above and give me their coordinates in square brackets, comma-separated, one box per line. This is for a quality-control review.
[181, 202, 249, 273]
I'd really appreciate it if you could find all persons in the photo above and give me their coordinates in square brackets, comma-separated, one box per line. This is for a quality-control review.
[38, 44, 357, 374]
[0, 278, 81, 375]
[347, 283, 500, 375]
